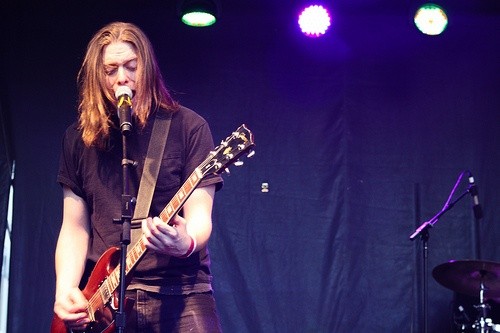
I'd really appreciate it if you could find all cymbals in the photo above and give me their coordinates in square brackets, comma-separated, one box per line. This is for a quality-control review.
[432, 260, 500, 296]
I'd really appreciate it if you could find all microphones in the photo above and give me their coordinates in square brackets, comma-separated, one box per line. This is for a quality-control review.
[115, 86, 133, 135]
[468, 170, 484, 220]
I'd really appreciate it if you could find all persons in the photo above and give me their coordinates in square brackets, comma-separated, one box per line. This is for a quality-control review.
[52, 21, 224, 333]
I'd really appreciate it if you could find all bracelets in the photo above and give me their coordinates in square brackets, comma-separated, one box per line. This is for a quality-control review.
[176, 236, 197, 259]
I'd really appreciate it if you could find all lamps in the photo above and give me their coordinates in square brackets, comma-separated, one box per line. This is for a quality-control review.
[180, 0, 217, 28]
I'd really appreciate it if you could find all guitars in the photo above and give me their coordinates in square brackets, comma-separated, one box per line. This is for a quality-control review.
[51, 124, 255, 333]
